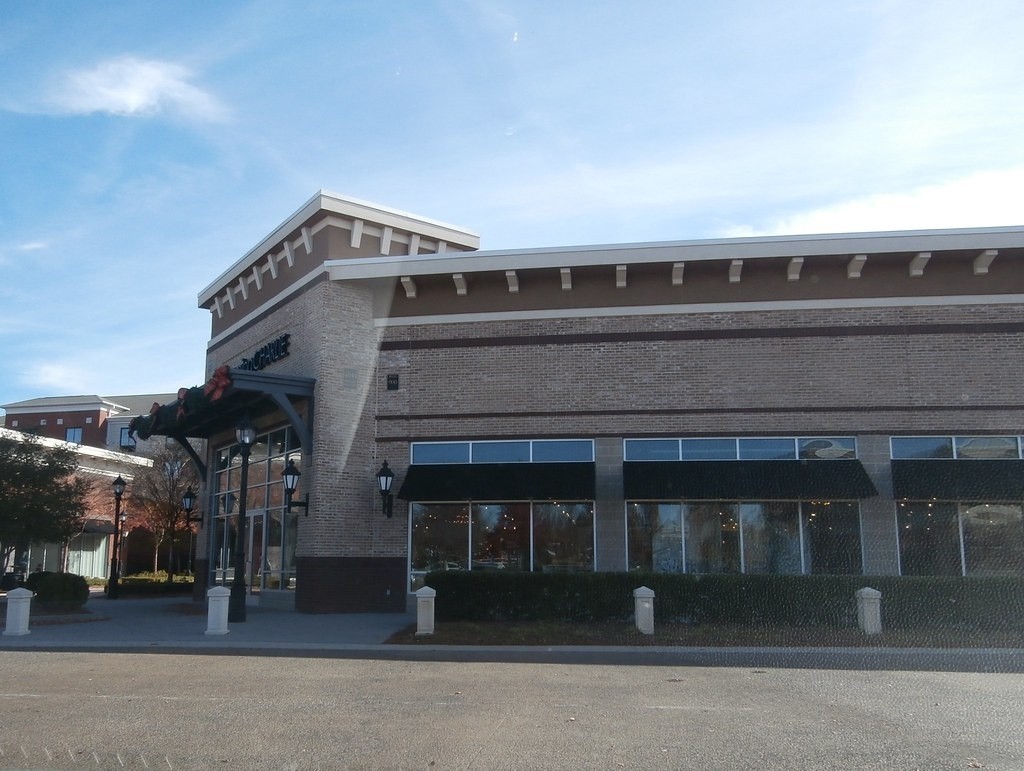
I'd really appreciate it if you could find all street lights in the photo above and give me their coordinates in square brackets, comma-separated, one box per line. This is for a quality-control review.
[105, 472, 128, 600]
[226, 420, 258, 623]
[118, 511, 129, 585]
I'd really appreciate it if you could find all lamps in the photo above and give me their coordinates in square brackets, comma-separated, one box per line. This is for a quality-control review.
[181, 485, 203, 529]
[280, 458, 309, 516]
[377, 460, 393, 518]
